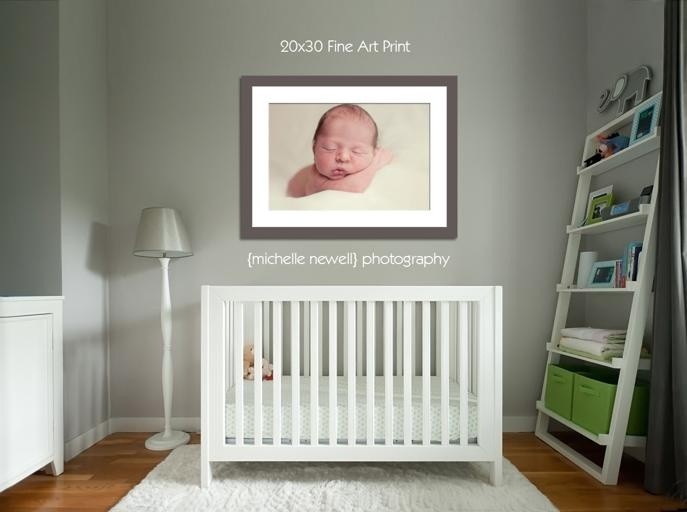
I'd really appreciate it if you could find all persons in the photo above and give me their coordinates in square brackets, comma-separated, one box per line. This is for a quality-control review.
[287, 104, 393, 198]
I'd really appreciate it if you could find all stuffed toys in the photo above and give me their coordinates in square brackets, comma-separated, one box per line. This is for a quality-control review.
[243, 343, 273, 380]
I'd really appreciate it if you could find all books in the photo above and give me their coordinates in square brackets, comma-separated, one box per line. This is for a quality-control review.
[616, 243, 642, 288]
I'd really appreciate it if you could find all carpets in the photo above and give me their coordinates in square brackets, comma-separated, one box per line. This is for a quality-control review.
[105, 441, 560, 512]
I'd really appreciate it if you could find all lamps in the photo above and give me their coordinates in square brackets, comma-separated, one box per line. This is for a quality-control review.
[130, 206, 195, 453]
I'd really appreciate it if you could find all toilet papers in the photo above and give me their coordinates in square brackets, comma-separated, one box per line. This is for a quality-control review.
[576, 252, 598, 288]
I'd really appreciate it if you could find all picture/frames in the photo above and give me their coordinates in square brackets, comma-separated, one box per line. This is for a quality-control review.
[238, 73, 459, 242]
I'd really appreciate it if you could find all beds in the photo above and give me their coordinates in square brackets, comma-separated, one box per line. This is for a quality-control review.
[198, 281, 506, 488]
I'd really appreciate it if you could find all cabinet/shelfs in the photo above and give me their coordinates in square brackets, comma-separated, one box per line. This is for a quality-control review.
[531, 90, 664, 488]
